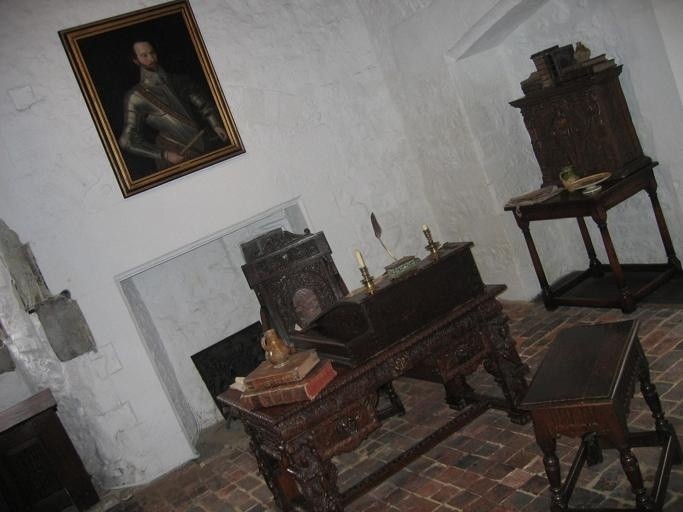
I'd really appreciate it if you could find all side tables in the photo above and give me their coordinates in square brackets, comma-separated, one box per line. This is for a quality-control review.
[517, 319, 683, 512]
[504, 161, 683, 314]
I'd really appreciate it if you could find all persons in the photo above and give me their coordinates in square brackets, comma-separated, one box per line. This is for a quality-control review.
[116, 34, 230, 166]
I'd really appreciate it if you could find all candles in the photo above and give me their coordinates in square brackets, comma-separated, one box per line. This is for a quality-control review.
[355, 249, 365, 267]
[421, 224, 429, 231]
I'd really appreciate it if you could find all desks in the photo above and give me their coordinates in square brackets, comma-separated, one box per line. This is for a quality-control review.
[216, 283, 532, 512]
[0, 387, 101, 512]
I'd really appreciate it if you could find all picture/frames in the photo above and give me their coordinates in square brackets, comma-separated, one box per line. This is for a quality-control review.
[57, 0, 246, 198]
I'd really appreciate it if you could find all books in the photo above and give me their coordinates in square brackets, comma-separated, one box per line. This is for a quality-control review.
[505, 183, 565, 207]
[240, 358, 341, 411]
[241, 345, 320, 393]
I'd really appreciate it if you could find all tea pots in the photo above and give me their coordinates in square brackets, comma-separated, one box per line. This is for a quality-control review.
[260, 328, 290, 369]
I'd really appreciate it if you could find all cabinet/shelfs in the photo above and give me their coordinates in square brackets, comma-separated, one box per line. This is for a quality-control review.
[241, 227, 350, 347]
[508, 63, 652, 189]
